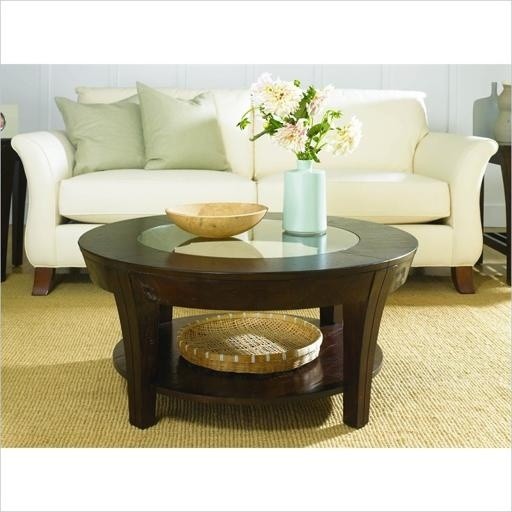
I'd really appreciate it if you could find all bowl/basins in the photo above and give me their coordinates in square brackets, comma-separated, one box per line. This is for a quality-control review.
[163, 234, 268, 272]
[163, 201, 269, 239]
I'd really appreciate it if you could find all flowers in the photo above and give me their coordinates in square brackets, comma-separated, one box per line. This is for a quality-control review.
[235, 70, 358, 166]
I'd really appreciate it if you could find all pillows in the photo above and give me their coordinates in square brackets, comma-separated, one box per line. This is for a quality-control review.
[133, 81, 233, 176]
[50, 90, 145, 177]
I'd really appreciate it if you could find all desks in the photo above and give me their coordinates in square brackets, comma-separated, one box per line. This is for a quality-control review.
[2, 138, 28, 284]
[475, 138, 511, 288]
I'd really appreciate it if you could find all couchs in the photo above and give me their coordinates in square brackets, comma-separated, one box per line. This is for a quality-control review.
[9, 86, 501, 299]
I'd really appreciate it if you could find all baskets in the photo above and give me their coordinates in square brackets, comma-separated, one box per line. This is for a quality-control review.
[176, 311, 325, 374]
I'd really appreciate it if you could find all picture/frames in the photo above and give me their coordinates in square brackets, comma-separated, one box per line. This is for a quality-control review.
[279, 158, 333, 237]
